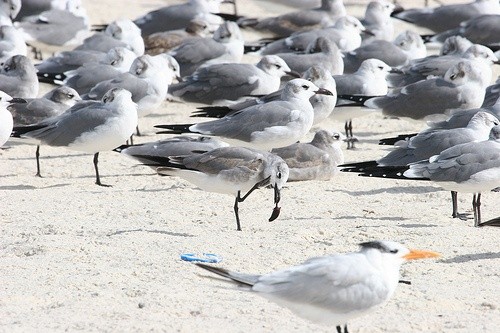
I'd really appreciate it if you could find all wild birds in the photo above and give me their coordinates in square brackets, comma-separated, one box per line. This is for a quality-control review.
[0, 0, 500, 231]
[190, 240, 442, 333]
